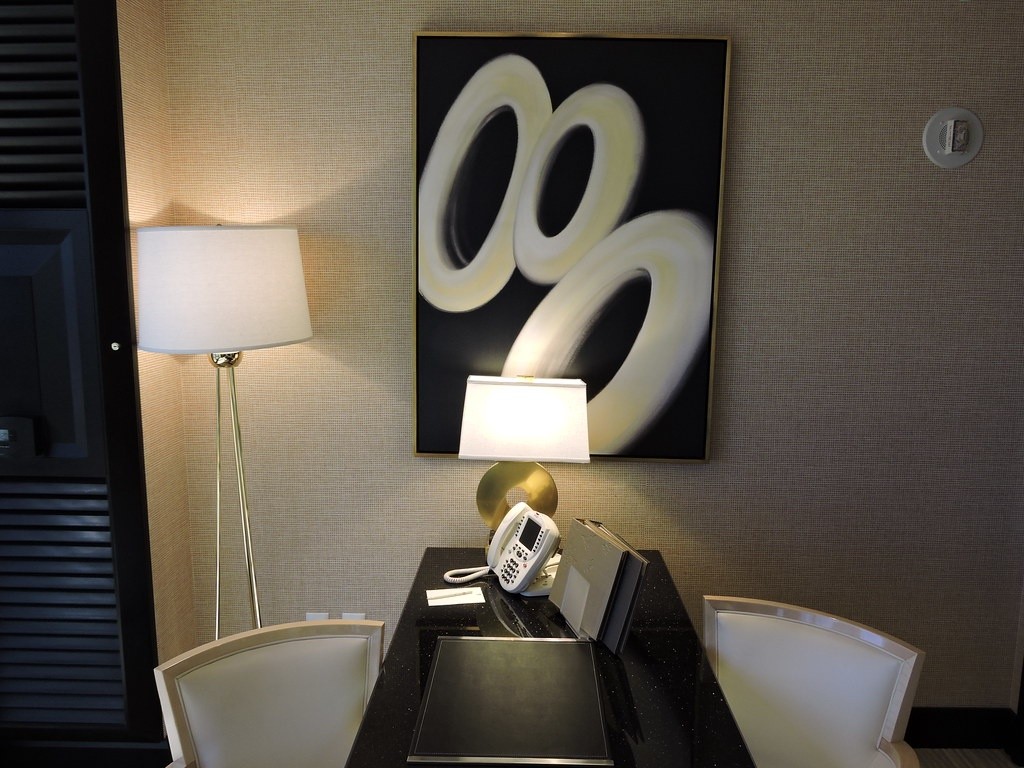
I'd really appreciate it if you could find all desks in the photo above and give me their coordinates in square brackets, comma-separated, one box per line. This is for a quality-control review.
[343, 548, 755, 767]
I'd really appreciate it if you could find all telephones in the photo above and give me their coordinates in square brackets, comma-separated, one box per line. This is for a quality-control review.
[485, 500, 562, 594]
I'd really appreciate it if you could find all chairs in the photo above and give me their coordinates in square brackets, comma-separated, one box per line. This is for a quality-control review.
[155, 619, 384, 767]
[702, 594, 926, 768]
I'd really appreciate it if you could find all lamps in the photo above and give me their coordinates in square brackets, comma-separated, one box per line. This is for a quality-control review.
[137, 224, 313, 641]
[458, 374, 590, 558]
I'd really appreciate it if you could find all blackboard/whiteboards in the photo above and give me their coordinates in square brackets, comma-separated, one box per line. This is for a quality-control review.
[407, 635, 614, 766]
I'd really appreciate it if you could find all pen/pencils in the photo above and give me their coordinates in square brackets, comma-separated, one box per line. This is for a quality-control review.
[427, 590, 473, 601]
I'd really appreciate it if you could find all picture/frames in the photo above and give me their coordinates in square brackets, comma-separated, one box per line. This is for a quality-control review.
[408, 34, 732, 463]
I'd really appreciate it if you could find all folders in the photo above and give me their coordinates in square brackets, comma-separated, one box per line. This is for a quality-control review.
[583, 519, 650, 655]
[548, 516, 629, 641]
